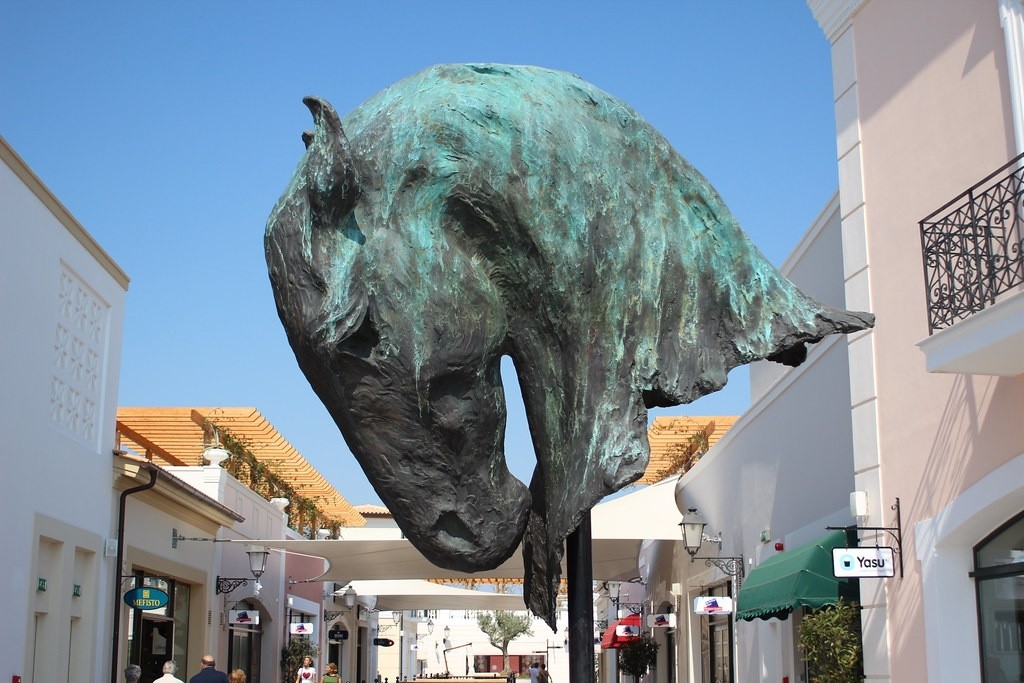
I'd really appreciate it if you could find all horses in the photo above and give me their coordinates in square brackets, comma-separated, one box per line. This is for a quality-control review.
[263, 61, 876, 634]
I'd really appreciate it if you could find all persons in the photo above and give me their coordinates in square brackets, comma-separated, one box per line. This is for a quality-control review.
[122, 665, 142, 683]
[321, 662, 342, 683]
[152, 659, 185, 683]
[226, 669, 246, 683]
[530, 663, 540, 683]
[189, 655, 229, 683]
[537, 664, 552, 683]
[295, 657, 316, 683]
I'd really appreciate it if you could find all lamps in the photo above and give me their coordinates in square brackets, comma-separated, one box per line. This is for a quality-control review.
[427, 620, 434, 635]
[392, 610, 402, 625]
[342, 585, 357, 610]
[245, 537, 271, 583]
[676, 505, 709, 564]
[606, 580, 622, 606]
[443, 625, 450, 638]
